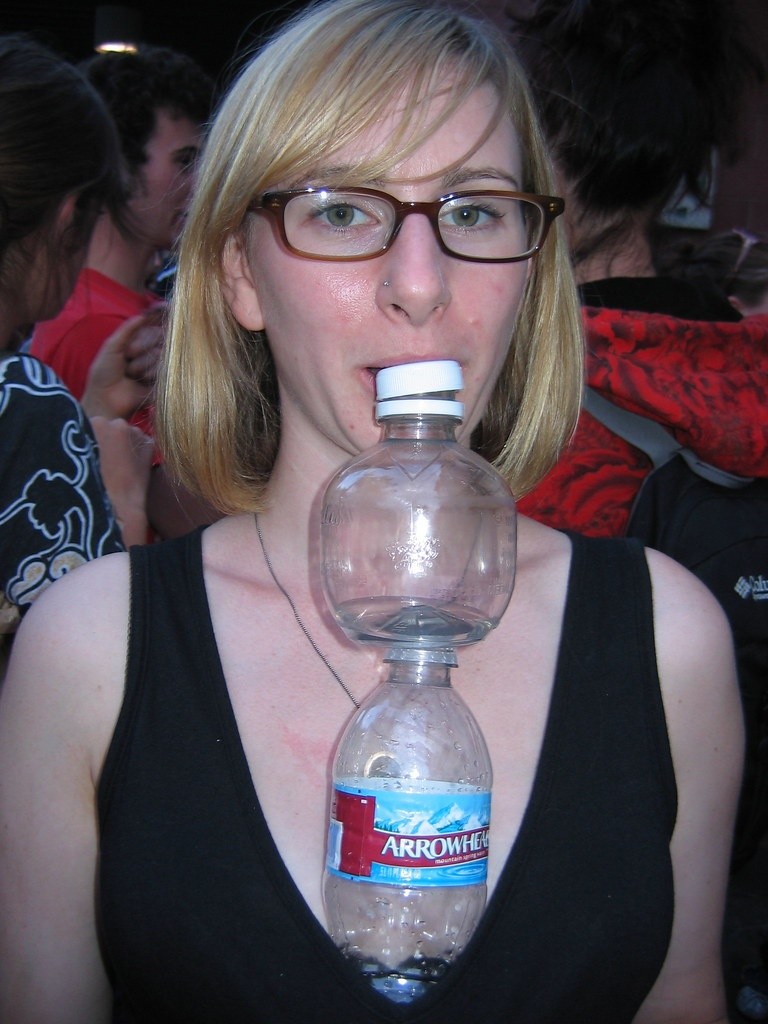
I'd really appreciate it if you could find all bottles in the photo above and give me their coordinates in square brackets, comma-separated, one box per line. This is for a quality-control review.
[317, 360, 518, 649]
[322, 651, 495, 1009]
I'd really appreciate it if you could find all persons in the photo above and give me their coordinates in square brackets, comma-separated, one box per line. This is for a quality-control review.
[0, 0, 768, 1024]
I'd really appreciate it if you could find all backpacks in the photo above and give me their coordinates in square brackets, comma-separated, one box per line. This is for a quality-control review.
[577, 382, 768, 872]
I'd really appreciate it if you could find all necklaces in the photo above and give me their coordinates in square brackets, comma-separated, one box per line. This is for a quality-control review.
[254, 511, 482, 748]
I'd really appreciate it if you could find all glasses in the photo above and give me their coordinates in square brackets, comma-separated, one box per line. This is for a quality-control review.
[246, 187, 565, 263]
[722, 226, 761, 294]
